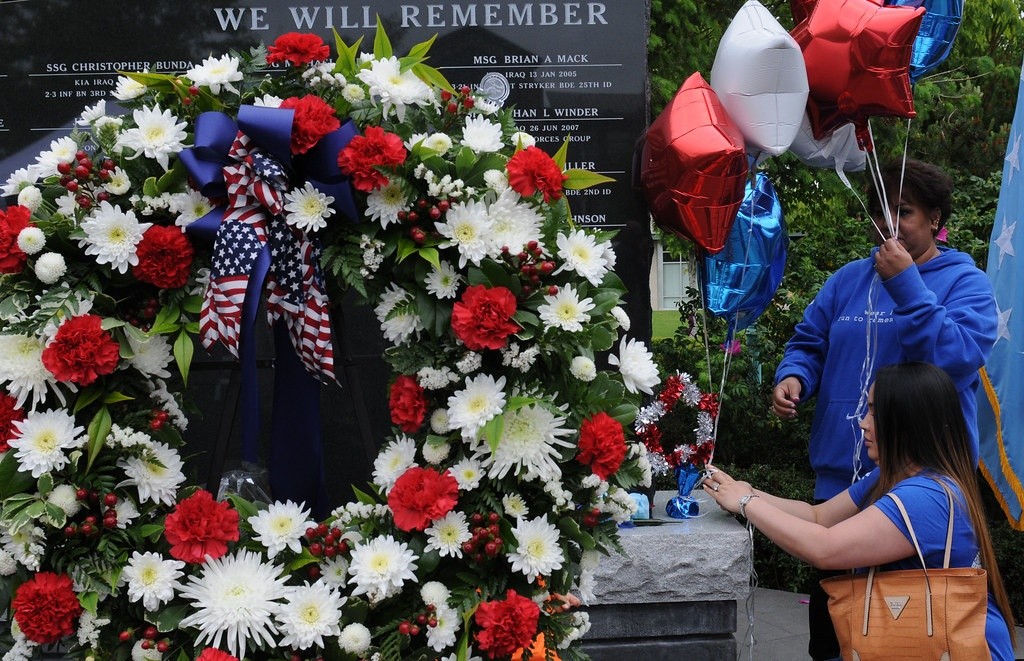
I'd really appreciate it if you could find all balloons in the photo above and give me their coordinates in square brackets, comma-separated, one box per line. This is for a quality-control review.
[793, 0, 886, 22]
[786, 104, 870, 172]
[712, 0, 809, 157]
[881, 0, 968, 90]
[641, 72, 749, 255]
[784, 0, 928, 143]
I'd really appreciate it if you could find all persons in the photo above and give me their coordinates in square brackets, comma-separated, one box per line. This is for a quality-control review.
[702, 360, 1018, 661]
[770, 155, 1000, 661]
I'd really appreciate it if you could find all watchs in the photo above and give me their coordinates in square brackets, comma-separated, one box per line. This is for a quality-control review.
[740, 494, 759, 519]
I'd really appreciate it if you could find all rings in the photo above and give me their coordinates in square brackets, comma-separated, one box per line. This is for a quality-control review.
[771, 406, 775, 412]
[713, 482, 719, 491]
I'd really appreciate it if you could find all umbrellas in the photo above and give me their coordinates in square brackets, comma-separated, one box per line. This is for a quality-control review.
[692, 172, 791, 347]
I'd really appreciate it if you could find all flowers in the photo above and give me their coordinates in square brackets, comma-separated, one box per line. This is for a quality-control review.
[0, 14, 668, 661]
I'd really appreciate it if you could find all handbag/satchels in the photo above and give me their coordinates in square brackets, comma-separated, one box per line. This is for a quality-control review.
[819, 567, 989, 661]
